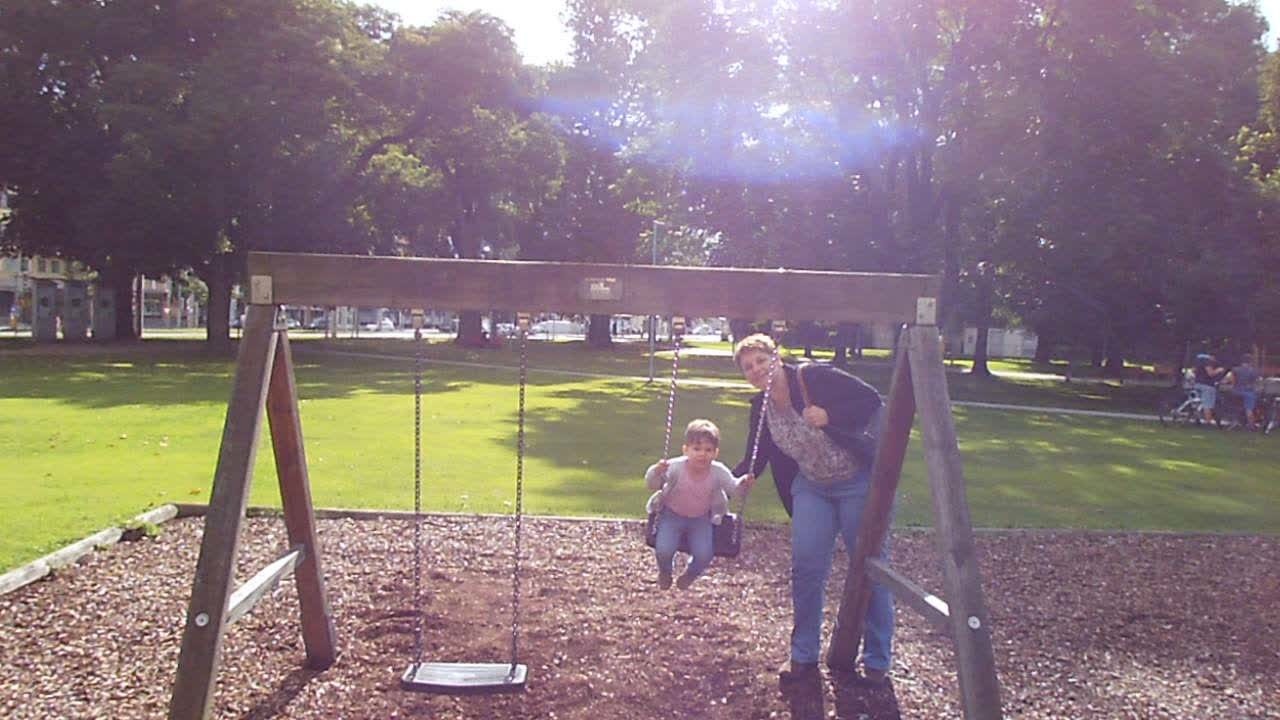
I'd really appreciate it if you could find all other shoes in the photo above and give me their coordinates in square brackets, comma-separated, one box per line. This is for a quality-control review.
[676, 566, 697, 589]
[777, 659, 817, 681]
[657, 569, 672, 589]
[856, 667, 886, 684]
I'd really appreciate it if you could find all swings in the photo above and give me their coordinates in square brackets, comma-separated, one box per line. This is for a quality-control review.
[647, 312, 788, 560]
[400, 308, 529, 695]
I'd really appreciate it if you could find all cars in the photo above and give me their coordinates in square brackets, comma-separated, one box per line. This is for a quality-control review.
[694, 325, 711, 336]
[287, 319, 295, 329]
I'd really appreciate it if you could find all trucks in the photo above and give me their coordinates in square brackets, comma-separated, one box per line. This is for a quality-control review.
[308, 318, 326, 329]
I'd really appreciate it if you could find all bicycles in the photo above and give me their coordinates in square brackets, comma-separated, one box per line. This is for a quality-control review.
[1159, 382, 1280, 436]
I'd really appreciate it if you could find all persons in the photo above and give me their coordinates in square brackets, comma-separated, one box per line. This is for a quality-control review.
[1231, 353, 1258, 418]
[646, 419, 755, 593]
[729, 333, 893, 688]
[1196, 348, 1224, 425]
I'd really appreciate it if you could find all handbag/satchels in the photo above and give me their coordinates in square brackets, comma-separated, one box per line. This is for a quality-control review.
[795, 364, 891, 444]
